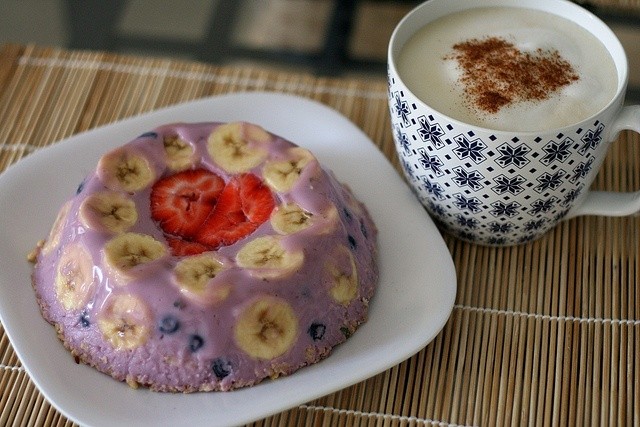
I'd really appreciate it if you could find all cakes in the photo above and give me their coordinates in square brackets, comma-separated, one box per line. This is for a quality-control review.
[27, 121, 379, 393]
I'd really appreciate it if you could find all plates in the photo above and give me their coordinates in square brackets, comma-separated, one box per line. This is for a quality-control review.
[0, 91, 457, 426]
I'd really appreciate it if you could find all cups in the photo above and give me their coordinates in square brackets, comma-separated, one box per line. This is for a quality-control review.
[384, 0, 640, 249]
